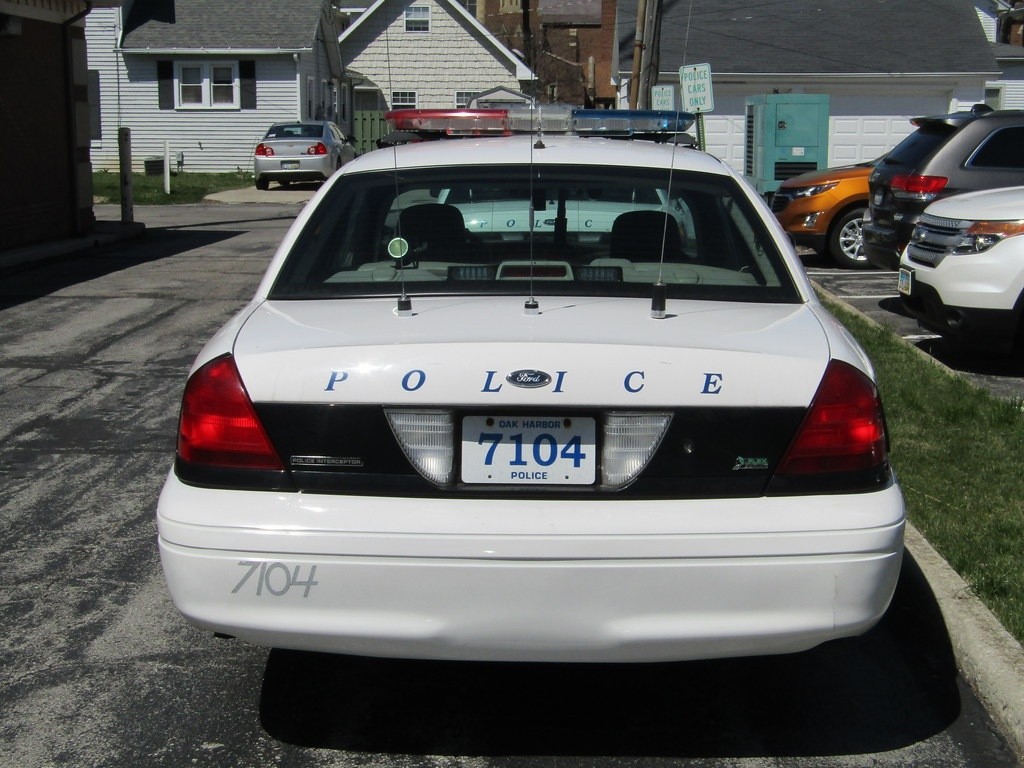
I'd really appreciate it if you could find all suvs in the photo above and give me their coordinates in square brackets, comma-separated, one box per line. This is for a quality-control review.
[857, 103, 1024, 272]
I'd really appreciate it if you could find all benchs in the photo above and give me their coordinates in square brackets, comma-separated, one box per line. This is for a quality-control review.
[323, 258, 756, 285]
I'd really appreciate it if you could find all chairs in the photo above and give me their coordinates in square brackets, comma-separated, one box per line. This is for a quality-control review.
[609, 210, 688, 263]
[396, 203, 466, 261]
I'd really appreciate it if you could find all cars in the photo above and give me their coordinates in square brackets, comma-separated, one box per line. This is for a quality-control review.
[898, 184, 1024, 349]
[157, 107, 903, 660]
[253, 120, 357, 190]
[769, 152, 890, 270]
